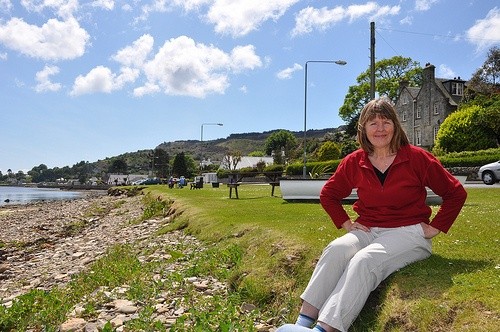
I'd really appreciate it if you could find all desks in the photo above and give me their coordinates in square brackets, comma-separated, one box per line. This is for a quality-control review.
[226, 171, 284, 199]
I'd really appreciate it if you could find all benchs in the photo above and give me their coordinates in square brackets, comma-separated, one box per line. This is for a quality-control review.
[190, 176, 203, 190]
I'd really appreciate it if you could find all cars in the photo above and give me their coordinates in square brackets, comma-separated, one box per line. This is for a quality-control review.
[131, 178, 162, 186]
[478, 160, 500, 186]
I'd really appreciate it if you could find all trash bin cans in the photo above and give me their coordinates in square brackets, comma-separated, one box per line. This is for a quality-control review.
[196, 176, 204, 188]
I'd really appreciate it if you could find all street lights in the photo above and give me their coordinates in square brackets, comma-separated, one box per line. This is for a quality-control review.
[200, 122, 223, 174]
[302, 60, 347, 179]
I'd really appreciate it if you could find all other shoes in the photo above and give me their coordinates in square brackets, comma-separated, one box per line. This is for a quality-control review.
[276, 324, 318, 332]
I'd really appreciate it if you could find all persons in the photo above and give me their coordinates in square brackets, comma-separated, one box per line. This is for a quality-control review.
[276, 99, 468, 332]
[168, 176, 186, 189]
